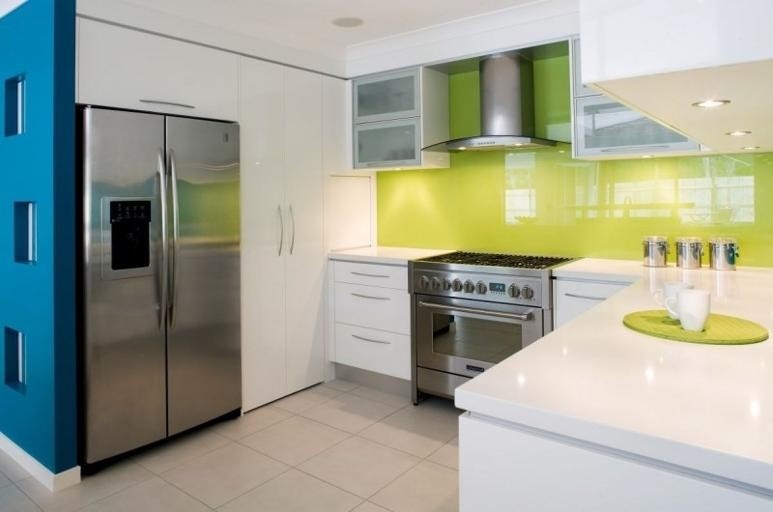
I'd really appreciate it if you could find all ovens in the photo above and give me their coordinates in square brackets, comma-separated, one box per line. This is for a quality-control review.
[412, 292, 542, 378]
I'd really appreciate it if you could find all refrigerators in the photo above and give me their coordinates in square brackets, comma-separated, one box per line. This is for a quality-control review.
[75, 100, 242, 466]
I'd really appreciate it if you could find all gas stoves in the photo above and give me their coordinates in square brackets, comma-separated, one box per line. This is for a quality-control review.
[407, 249, 580, 309]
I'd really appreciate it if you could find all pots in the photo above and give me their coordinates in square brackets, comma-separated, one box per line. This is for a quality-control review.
[641, 234, 740, 271]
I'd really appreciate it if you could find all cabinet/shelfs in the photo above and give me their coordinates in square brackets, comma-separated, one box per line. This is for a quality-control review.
[238, 53, 325, 416]
[75, 13, 239, 124]
[328, 244, 459, 380]
[351, 65, 450, 171]
[568, 35, 701, 161]
[551, 255, 644, 333]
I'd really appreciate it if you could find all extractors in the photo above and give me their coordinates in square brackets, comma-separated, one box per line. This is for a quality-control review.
[421, 56, 572, 155]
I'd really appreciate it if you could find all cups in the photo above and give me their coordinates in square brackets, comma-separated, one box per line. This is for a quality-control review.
[663, 287, 715, 335]
[651, 283, 691, 319]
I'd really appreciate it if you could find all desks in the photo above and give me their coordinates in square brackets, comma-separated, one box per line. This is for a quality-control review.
[454, 262, 773, 512]
